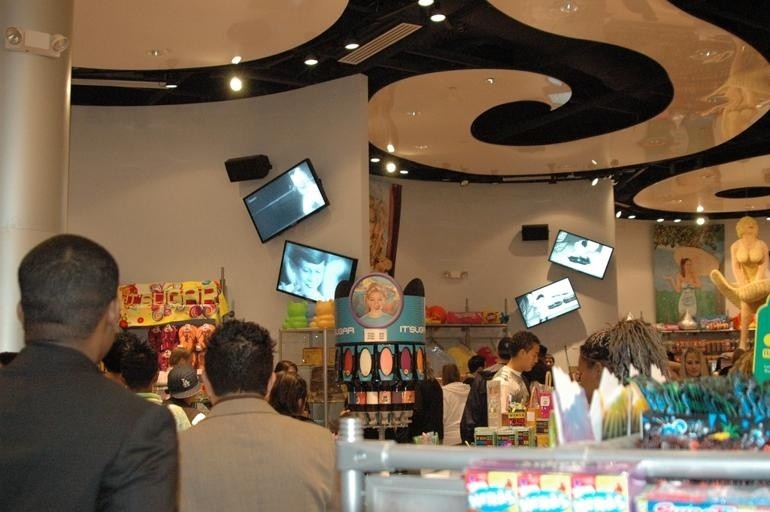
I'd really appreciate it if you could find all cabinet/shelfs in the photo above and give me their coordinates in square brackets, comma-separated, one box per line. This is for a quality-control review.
[656, 323, 754, 375]
[425, 294, 511, 354]
[279, 327, 344, 435]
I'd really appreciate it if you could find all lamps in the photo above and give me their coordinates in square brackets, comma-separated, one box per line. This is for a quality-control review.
[1, 27, 67, 60]
[442, 270, 468, 279]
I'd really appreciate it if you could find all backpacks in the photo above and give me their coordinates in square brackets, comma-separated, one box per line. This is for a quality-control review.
[460, 370, 531, 445]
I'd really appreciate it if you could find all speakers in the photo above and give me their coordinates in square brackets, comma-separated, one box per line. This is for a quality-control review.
[522, 224, 548, 240]
[225, 155, 269, 182]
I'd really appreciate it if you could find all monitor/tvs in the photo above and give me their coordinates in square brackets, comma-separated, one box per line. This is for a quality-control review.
[276, 240, 358, 302]
[548, 229, 614, 280]
[242, 158, 329, 243]
[515, 277, 581, 329]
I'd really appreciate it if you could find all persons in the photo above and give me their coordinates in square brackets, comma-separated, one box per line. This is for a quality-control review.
[668, 346, 729, 381]
[167, 319, 345, 511]
[366, 330, 554, 444]
[286, 245, 328, 301]
[0, 231, 180, 511]
[661, 258, 704, 319]
[578, 319, 671, 441]
[100, 332, 313, 432]
[358, 282, 393, 325]
[728, 216, 770, 351]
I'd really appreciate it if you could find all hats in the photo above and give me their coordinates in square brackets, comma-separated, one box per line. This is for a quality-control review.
[167, 363, 202, 399]
